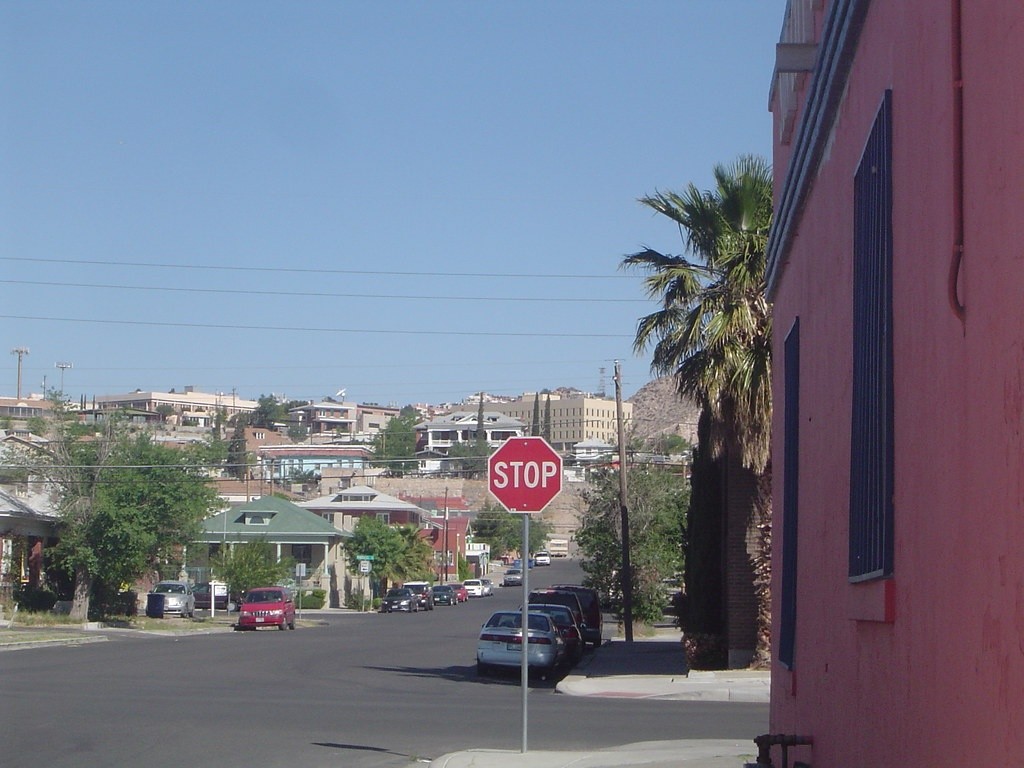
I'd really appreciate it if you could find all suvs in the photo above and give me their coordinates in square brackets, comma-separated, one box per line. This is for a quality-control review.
[503, 568, 522, 585]
[401, 580, 435, 611]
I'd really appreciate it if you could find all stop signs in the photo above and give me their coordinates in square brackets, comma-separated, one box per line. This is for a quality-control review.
[487, 436, 562, 513]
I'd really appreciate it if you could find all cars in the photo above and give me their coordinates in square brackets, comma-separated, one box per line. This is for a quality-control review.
[430, 578, 492, 606]
[239, 586, 297, 632]
[380, 587, 418, 614]
[476, 588, 589, 678]
[535, 552, 551, 565]
[146, 579, 243, 618]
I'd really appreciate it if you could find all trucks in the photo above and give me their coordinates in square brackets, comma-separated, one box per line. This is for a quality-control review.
[548, 538, 570, 558]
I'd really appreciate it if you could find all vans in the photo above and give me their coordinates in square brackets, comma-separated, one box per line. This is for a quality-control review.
[548, 583, 604, 647]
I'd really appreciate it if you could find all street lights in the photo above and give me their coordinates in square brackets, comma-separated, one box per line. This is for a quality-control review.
[8, 346, 30, 401]
[53, 361, 73, 392]
